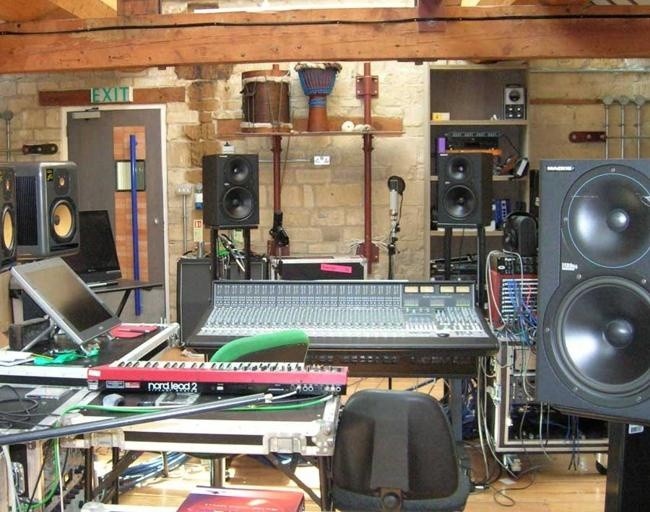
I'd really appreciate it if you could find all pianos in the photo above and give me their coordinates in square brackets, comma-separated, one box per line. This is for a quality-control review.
[87, 359, 349, 395]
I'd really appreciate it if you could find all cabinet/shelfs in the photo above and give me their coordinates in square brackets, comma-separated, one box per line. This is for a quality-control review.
[427, 64, 530, 285]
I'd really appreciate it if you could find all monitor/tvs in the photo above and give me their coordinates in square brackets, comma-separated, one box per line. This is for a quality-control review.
[63, 210, 122, 287]
[9, 255, 121, 345]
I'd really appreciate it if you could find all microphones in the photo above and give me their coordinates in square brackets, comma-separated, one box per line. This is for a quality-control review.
[387, 175, 406, 217]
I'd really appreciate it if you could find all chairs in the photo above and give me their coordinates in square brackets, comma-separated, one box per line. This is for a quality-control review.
[326, 386, 473, 511]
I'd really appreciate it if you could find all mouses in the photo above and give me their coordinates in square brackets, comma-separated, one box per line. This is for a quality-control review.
[102, 393, 126, 407]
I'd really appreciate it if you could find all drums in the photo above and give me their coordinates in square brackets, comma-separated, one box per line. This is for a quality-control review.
[242, 70, 292, 132]
[295, 63, 342, 131]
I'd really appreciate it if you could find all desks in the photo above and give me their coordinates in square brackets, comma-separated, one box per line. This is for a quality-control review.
[90, 277, 163, 317]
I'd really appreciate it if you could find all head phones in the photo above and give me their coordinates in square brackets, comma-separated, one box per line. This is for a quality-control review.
[504, 210, 538, 249]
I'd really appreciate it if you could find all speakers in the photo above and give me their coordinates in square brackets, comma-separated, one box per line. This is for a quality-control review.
[202, 154, 260, 228]
[535, 160, 650, 425]
[0, 167, 17, 272]
[436, 152, 492, 228]
[177, 259, 269, 353]
[14, 161, 80, 259]
